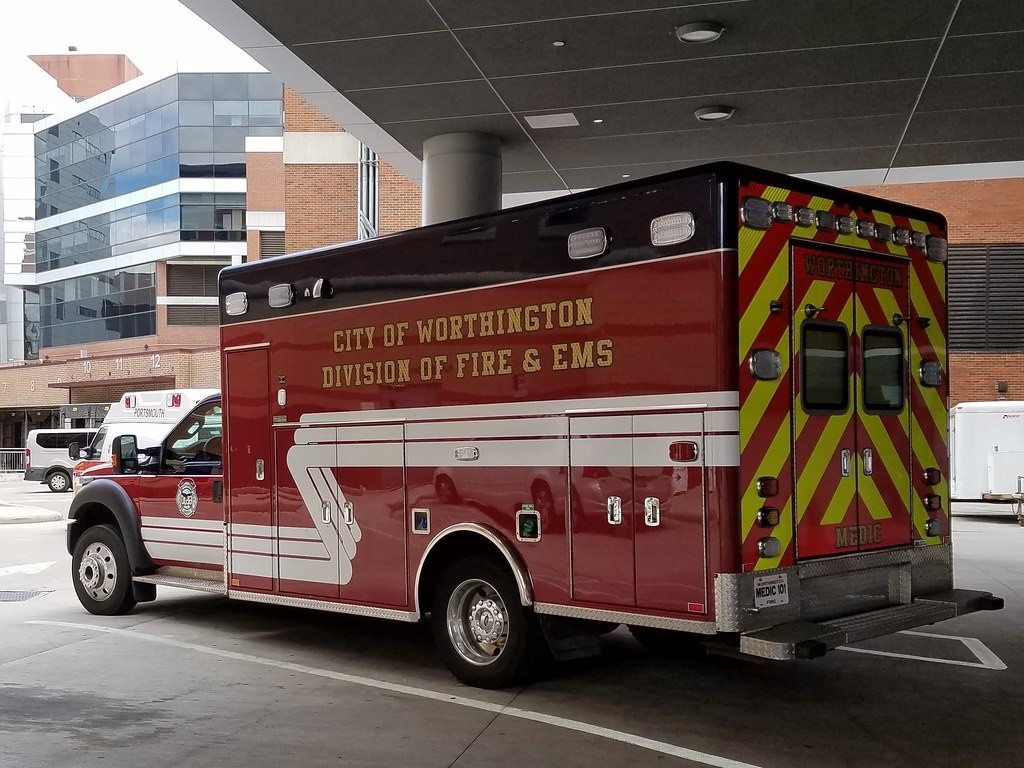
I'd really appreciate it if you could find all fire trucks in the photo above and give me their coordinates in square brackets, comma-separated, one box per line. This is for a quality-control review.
[66, 159, 1006, 694]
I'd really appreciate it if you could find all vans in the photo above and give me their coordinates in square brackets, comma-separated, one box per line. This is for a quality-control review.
[80, 387, 223, 467]
[23, 427, 100, 493]
